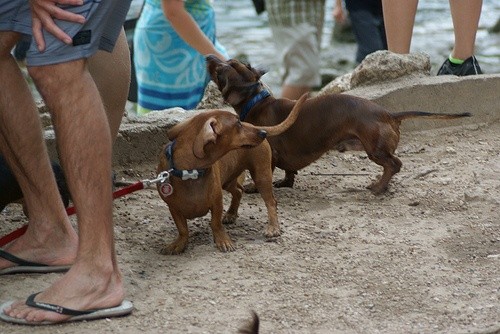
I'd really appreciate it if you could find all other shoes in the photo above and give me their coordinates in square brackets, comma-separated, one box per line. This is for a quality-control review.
[437, 57, 482, 76]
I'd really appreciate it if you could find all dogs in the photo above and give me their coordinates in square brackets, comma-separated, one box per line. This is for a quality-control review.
[205, 54, 403, 195]
[156, 91, 312, 256]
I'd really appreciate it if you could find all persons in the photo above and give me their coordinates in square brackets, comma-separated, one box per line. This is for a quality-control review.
[86, 0, 483, 144]
[0, 0, 132, 327]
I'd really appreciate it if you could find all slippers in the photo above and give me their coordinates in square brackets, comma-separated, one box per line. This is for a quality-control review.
[0, 250, 74, 275]
[1, 292, 134, 326]
